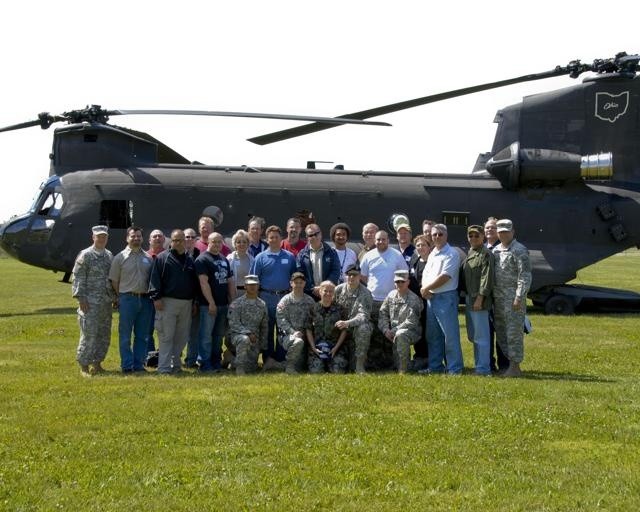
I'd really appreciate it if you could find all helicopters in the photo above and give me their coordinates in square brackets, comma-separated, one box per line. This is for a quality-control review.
[0, 51, 640, 315]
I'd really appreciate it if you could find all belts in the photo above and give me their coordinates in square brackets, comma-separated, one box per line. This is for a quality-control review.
[259, 287, 290, 295]
[127, 292, 148, 297]
[236, 286, 245, 289]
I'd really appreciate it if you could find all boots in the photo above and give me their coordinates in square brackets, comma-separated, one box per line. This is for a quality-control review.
[81, 361, 105, 377]
[235, 360, 299, 374]
[498, 361, 522, 376]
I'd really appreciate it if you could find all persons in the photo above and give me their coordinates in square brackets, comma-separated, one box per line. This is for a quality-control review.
[71, 226, 119, 380]
[481, 218, 511, 374]
[489, 218, 534, 378]
[146, 212, 464, 376]
[461, 224, 494, 377]
[109, 227, 154, 377]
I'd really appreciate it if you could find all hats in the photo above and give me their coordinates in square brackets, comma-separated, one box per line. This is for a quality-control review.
[397, 224, 412, 232]
[316, 343, 330, 359]
[345, 264, 361, 274]
[244, 275, 259, 284]
[92, 225, 108, 235]
[290, 272, 305, 280]
[467, 225, 484, 235]
[496, 219, 512, 232]
[394, 270, 409, 282]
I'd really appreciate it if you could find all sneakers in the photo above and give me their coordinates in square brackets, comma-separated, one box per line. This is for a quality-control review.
[418, 367, 432, 373]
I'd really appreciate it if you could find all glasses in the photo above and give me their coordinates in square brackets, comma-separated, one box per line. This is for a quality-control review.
[186, 236, 195, 238]
[431, 233, 444, 236]
[307, 231, 320, 237]
